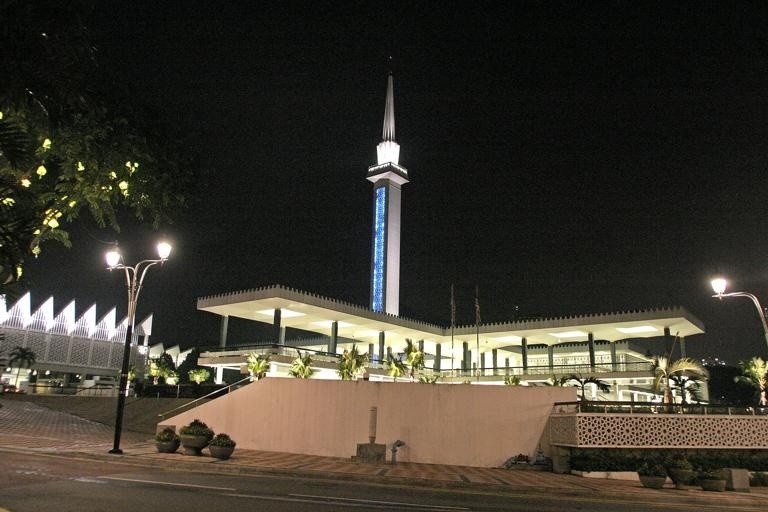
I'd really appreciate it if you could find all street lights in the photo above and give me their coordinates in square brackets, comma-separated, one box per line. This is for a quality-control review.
[101, 236, 174, 453]
[710, 276, 767, 347]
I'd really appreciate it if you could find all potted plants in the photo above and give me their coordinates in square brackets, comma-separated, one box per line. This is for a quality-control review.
[178, 418, 214, 458]
[636, 457, 667, 488]
[698, 467, 731, 491]
[153, 427, 180, 453]
[665, 459, 700, 490]
[206, 431, 237, 459]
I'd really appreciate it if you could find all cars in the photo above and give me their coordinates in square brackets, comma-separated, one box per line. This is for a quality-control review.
[3, 384, 19, 394]
